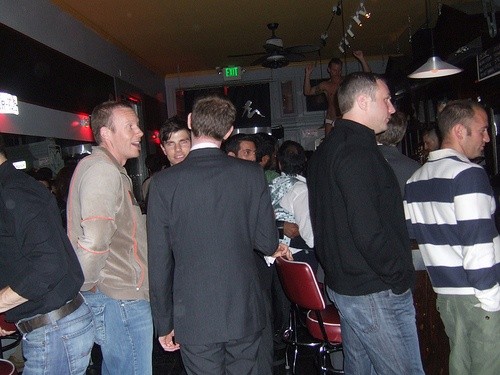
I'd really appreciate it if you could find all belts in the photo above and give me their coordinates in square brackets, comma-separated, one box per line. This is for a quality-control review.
[15, 292, 84, 333]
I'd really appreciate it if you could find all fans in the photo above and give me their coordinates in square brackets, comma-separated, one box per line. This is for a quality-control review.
[227, 22, 320, 69]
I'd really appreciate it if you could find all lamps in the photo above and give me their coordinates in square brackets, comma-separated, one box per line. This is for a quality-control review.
[347, 25, 356, 40]
[359, 3, 371, 18]
[407, 0, 463, 79]
[338, 42, 344, 53]
[353, 12, 362, 27]
[342, 34, 352, 49]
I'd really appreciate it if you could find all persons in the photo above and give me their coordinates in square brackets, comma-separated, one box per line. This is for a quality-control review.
[421, 122, 440, 153]
[24, 152, 89, 229]
[307, 71, 426, 375]
[253, 130, 315, 185]
[268, 140, 319, 277]
[402, 97, 500, 375]
[0, 137, 95, 375]
[66, 100, 154, 375]
[302, 50, 373, 137]
[225, 133, 258, 163]
[147, 94, 293, 374]
[141, 154, 163, 202]
[158, 115, 192, 169]
[374, 105, 424, 201]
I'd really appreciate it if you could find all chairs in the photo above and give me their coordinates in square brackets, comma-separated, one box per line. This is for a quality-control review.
[274, 256, 344, 375]
